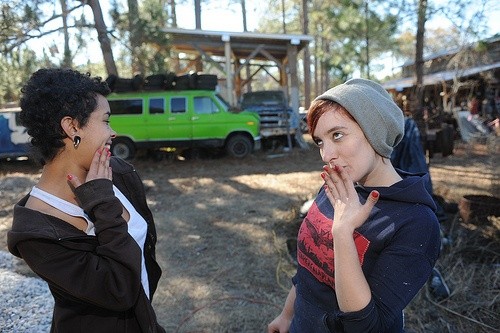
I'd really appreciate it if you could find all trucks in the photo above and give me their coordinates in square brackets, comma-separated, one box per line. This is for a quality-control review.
[0, 106, 37, 162]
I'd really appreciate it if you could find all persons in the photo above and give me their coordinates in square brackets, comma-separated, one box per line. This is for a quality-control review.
[392, 92, 500, 118]
[266, 78, 442, 333]
[6, 67, 166, 333]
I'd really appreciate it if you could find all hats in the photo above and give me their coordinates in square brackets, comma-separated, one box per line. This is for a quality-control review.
[313, 77, 406, 160]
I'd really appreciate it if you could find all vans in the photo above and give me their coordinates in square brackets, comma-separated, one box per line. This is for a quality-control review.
[104, 73, 261, 164]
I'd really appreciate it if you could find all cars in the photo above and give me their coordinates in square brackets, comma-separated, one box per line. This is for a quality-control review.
[239, 89, 298, 148]
